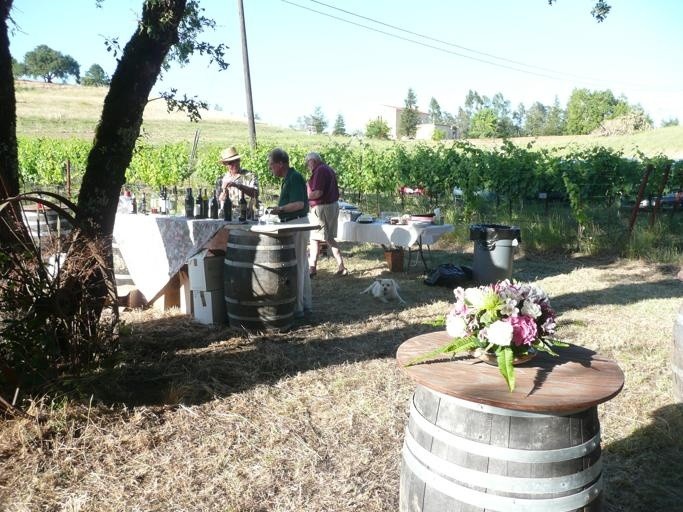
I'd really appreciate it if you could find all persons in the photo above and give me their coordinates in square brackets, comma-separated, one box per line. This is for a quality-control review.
[305, 153, 347, 278]
[264, 148, 312, 319]
[217, 146, 258, 209]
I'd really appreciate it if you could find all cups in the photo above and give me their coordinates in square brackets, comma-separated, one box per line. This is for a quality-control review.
[432, 216, 443, 225]
[384, 214, 407, 225]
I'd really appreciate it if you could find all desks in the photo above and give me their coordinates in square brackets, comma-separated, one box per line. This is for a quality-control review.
[262, 211, 454, 272]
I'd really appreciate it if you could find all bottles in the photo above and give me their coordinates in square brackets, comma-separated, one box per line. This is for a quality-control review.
[121, 186, 258, 224]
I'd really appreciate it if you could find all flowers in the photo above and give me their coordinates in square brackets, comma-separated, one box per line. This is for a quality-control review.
[404, 278, 570, 392]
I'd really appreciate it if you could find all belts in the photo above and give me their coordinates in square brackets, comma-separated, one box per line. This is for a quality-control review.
[280, 215, 307, 223]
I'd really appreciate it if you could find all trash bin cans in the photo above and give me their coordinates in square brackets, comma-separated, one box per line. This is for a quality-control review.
[472, 224, 520, 284]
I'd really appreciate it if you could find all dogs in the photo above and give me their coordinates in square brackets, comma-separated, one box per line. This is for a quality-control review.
[360, 278, 407, 305]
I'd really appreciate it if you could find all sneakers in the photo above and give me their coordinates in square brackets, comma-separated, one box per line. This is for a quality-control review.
[332, 267, 348, 278]
[304, 308, 314, 316]
[309, 266, 318, 278]
[294, 310, 305, 319]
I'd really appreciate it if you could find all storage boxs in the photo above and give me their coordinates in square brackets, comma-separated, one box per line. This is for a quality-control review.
[187, 255, 225, 292]
[384, 248, 404, 274]
[126, 262, 192, 316]
[192, 288, 226, 324]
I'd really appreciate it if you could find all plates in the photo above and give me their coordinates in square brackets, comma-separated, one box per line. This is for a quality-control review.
[358, 221, 372, 223]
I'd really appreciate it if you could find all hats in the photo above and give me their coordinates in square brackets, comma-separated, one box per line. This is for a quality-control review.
[218, 146, 243, 162]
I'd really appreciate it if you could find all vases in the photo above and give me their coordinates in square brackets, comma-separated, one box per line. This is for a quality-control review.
[475, 348, 539, 366]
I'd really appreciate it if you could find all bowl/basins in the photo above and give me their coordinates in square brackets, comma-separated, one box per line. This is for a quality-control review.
[342, 211, 361, 222]
